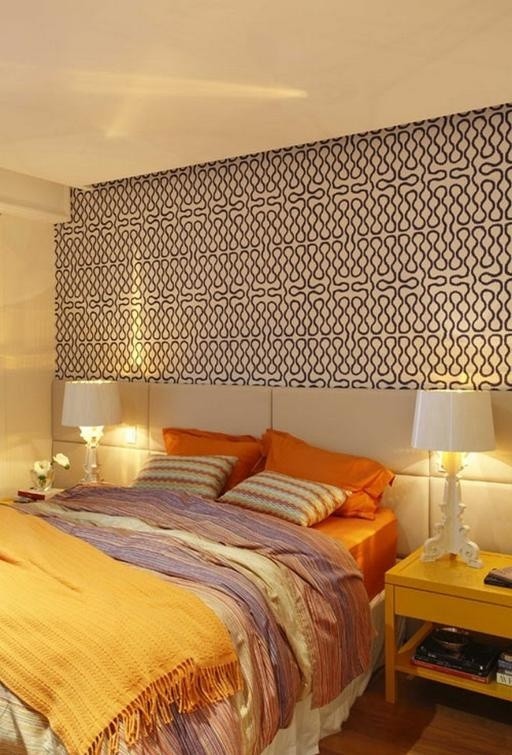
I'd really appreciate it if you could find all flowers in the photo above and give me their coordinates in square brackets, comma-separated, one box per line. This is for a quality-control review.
[34, 453, 71, 486]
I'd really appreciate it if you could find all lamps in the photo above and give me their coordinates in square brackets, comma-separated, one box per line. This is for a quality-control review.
[411, 390, 496, 569]
[61, 381, 122, 482]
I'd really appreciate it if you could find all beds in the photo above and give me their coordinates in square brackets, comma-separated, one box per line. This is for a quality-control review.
[0, 381, 512, 755]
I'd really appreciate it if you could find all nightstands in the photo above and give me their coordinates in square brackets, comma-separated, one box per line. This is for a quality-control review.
[384, 547, 512, 704]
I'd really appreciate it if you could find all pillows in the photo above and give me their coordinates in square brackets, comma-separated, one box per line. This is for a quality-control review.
[131, 427, 395, 527]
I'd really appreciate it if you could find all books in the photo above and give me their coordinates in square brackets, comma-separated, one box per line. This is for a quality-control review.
[496, 652, 511, 688]
[410, 654, 498, 685]
[484, 574, 512, 590]
[13, 495, 32, 504]
[414, 626, 500, 677]
[16, 489, 58, 502]
[489, 565, 512, 584]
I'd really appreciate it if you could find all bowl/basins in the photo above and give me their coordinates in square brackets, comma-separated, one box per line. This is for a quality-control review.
[432, 626, 476, 651]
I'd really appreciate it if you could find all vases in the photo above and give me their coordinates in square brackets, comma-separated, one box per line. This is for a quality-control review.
[31, 472, 55, 491]
